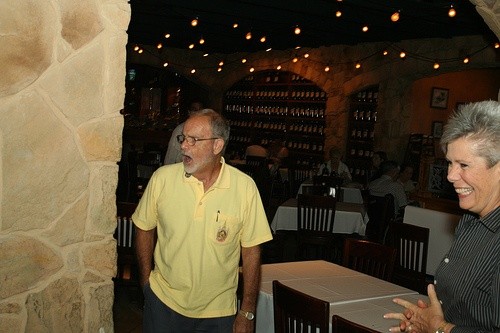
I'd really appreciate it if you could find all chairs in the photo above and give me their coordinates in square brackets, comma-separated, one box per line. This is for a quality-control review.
[114, 156, 434, 333]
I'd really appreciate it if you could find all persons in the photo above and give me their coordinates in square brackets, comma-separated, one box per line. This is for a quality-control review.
[130, 108, 275, 333]
[383, 100, 500, 332]
[316, 146, 353, 185]
[234, 144, 289, 211]
[164, 98, 215, 166]
[366, 151, 420, 221]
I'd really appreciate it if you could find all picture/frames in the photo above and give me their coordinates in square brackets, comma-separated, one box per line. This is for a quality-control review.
[432, 120, 443, 140]
[430, 87, 449, 111]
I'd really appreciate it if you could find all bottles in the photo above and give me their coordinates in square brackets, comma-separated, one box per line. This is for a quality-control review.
[146, 104, 154, 120]
[224, 74, 380, 186]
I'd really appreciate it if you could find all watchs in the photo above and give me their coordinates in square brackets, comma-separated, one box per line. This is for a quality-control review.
[239, 310, 254, 321]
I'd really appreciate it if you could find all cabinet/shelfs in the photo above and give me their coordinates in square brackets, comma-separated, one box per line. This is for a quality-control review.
[222, 70, 379, 174]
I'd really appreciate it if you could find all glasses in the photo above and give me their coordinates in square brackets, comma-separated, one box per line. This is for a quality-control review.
[176, 134, 219, 145]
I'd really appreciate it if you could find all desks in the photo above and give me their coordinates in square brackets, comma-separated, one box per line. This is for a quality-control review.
[269, 199, 370, 236]
[237, 261, 427, 333]
[229, 159, 272, 169]
[296, 180, 363, 204]
